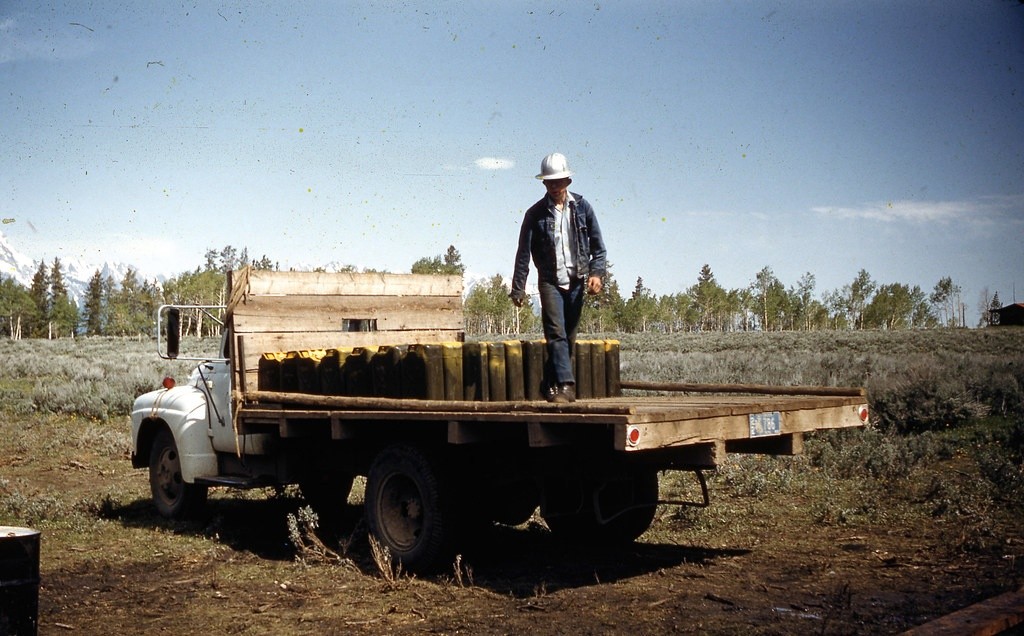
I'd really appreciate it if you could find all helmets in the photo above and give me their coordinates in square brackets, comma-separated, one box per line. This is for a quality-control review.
[535, 153, 576, 180]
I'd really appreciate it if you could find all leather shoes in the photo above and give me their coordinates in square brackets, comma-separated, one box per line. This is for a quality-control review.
[540, 381, 560, 402]
[553, 383, 575, 403]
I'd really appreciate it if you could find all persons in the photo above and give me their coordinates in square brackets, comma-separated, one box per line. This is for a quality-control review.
[508, 153, 607, 402]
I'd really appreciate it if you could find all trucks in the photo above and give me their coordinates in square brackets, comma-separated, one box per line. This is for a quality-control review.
[128, 267, 869, 579]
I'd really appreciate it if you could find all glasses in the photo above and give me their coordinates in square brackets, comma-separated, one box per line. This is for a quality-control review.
[543, 178, 570, 187]
